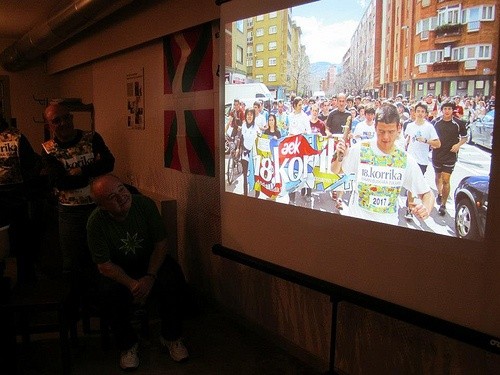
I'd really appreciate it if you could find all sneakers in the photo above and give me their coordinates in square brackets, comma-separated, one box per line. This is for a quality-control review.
[336, 200, 342, 210]
[406, 207, 414, 219]
[160, 334, 189, 361]
[121, 347, 139, 368]
[332, 192, 337, 201]
[438, 205, 445, 214]
[436, 194, 443, 205]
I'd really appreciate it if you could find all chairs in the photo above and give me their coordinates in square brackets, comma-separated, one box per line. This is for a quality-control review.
[1, 225, 153, 375]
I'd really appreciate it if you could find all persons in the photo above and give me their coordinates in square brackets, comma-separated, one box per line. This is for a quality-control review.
[331, 102, 436, 225]
[86, 174, 190, 370]
[0, 115, 37, 282]
[403, 103, 441, 221]
[226, 90, 495, 215]
[37, 103, 115, 279]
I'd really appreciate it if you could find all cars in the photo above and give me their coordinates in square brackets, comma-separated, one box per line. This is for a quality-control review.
[466, 110, 494, 151]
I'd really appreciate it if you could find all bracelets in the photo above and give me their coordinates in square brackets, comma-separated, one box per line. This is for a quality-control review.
[146, 272, 157, 282]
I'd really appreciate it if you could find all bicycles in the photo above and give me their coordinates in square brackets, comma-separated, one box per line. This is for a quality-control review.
[226, 134, 242, 183]
